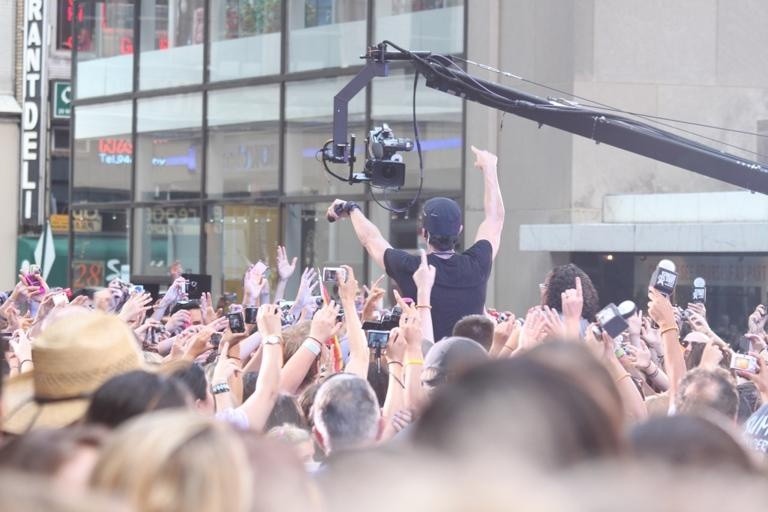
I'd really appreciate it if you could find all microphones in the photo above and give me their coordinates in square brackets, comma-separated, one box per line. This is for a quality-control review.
[592, 300, 636, 340]
[691, 277, 707, 303]
[327, 204, 348, 222]
[650, 258, 679, 330]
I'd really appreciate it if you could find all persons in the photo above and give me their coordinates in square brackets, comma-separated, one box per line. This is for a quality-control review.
[325, 145, 505, 342]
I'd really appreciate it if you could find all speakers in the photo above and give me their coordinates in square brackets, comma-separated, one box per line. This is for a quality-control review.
[137, 283, 159, 317]
[182, 273, 211, 298]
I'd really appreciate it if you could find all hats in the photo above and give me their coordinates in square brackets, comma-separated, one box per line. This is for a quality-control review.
[0, 311, 192, 435]
[419, 198, 462, 237]
[421, 337, 494, 393]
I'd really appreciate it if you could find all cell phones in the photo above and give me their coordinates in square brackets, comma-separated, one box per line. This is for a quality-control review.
[26, 275, 45, 293]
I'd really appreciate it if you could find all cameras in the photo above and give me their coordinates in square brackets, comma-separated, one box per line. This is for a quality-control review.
[178, 281, 186, 295]
[245, 308, 277, 324]
[367, 330, 391, 349]
[322, 267, 346, 283]
[127, 285, 143, 295]
[729, 352, 756, 374]
[0, 334, 19, 352]
[52, 292, 70, 306]
[761, 305, 768, 316]
[228, 312, 245, 333]
